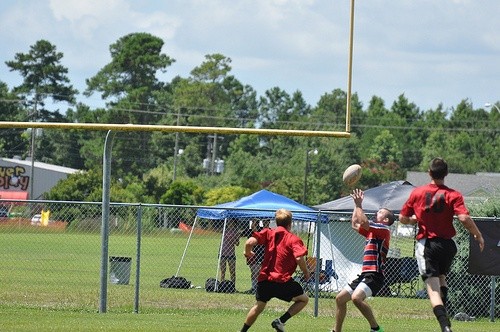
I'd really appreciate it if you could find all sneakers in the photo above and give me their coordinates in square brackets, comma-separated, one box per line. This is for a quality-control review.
[370, 325, 384, 332]
[272, 318, 284, 332]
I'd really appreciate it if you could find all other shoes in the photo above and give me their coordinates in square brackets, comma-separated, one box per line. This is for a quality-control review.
[444, 326, 451, 332]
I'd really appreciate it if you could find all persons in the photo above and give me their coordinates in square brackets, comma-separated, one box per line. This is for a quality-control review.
[218, 219, 239, 287]
[239, 209, 311, 332]
[245, 219, 272, 294]
[330, 188, 395, 332]
[399, 157, 484, 332]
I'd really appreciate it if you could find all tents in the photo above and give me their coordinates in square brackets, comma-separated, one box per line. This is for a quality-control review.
[175, 180, 418, 294]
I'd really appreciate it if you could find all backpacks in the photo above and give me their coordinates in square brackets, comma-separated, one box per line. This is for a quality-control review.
[160, 276, 191, 289]
[205, 278, 220, 292]
[218, 280, 235, 293]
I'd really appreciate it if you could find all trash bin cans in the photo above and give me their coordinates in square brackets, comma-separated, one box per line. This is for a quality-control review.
[108, 255, 132, 285]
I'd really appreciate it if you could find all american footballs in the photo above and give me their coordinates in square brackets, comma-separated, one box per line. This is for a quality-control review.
[343, 164, 362, 185]
